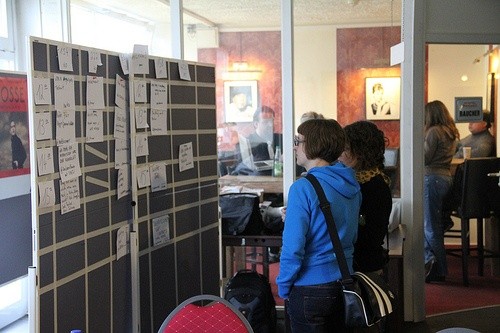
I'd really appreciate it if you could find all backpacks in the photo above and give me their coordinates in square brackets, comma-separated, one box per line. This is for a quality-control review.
[224, 269, 277, 333]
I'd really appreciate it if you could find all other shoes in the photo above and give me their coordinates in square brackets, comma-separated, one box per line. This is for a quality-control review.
[424, 258, 447, 282]
[268, 247, 280, 263]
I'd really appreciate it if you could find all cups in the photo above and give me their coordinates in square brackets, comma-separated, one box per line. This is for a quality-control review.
[462, 146, 471, 159]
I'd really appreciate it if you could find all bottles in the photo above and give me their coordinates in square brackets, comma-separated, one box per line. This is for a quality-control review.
[273, 145, 282, 177]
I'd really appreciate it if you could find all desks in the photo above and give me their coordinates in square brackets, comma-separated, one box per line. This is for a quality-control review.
[220, 157, 307, 194]
[385, 156, 495, 275]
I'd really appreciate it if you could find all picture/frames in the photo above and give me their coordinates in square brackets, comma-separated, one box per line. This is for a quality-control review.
[225, 80, 259, 123]
[365, 76, 402, 121]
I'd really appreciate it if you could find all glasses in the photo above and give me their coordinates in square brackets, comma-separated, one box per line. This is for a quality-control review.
[294, 136, 305, 146]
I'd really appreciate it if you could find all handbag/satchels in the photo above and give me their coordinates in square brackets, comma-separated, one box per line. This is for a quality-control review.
[219, 192, 264, 237]
[341, 271, 400, 333]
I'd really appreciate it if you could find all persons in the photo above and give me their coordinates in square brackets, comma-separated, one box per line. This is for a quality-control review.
[444, 110, 497, 234]
[275, 118, 392, 333]
[234, 105, 326, 264]
[230, 87, 253, 115]
[10, 120, 26, 170]
[423, 100, 456, 284]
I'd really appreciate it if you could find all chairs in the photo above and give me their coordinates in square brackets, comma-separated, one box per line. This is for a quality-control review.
[158, 137, 500, 333]
